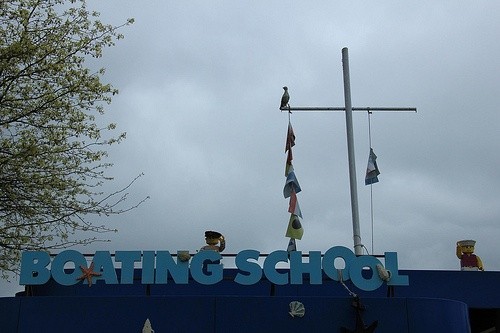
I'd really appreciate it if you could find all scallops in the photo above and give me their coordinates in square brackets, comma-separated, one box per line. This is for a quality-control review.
[288, 301, 305, 318]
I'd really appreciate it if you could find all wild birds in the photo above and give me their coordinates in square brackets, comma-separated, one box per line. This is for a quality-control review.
[280, 85, 290, 108]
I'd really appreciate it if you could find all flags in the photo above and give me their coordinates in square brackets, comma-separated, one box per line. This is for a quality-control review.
[282, 120, 304, 241]
[364, 147, 381, 186]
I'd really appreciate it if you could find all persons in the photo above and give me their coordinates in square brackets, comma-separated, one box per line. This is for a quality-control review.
[455, 239, 485, 271]
[199, 230, 226, 254]
